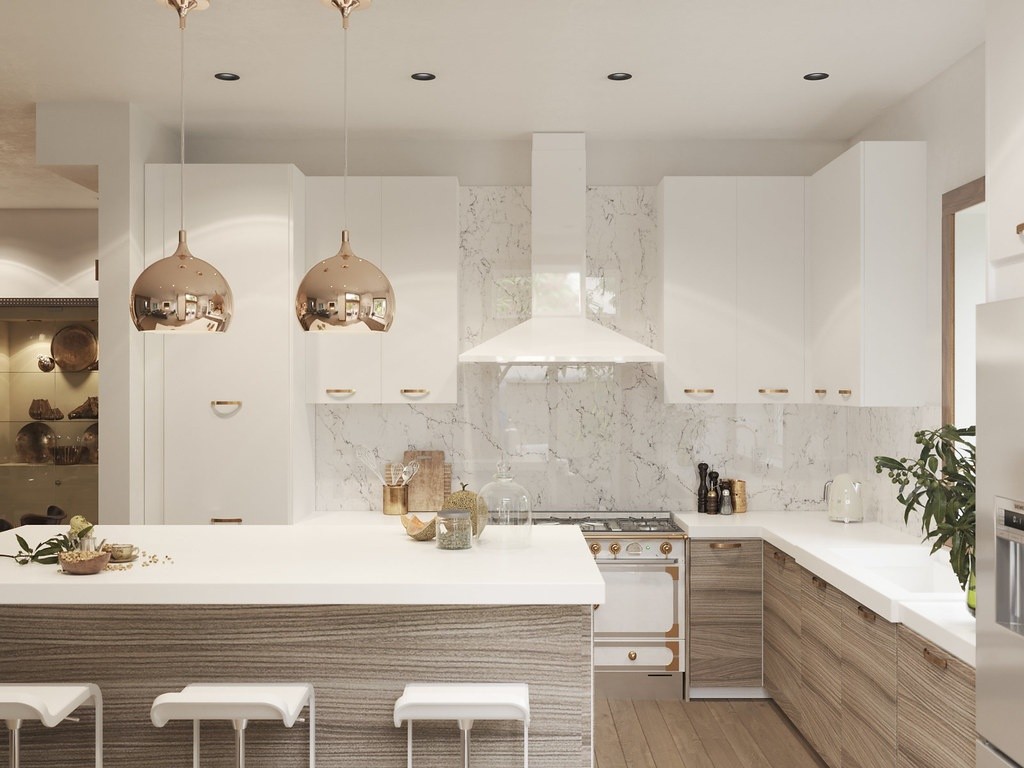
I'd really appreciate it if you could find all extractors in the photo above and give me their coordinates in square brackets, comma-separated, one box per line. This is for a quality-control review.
[458, 131, 662, 363]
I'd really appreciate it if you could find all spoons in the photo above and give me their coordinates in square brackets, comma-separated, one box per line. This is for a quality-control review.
[390, 460, 419, 486]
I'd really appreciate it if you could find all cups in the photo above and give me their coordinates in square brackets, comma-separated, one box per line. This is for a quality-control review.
[382, 484, 409, 515]
[731, 479, 748, 513]
[111, 543, 139, 560]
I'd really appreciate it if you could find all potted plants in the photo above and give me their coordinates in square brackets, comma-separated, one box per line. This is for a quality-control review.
[874, 423, 976, 614]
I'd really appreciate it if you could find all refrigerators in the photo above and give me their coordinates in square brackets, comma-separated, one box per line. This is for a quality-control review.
[975, 294, 1024, 768]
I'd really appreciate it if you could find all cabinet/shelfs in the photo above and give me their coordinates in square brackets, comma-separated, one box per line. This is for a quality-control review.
[684, 537, 976, 767]
[306, 177, 462, 406]
[0, 297, 98, 532]
[663, 140, 929, 405]
[144, 164, 317, 523]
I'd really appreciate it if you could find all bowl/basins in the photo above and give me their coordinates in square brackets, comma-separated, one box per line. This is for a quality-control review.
[49, 446, 84, 465]
[58, 551, 112, 575]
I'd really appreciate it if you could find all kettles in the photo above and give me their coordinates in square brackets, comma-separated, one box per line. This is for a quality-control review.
[823, 474, 864, 523]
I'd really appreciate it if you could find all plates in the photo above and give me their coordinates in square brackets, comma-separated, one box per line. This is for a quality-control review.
[109, 555, 139, 563]
[50, 324, 98, 372]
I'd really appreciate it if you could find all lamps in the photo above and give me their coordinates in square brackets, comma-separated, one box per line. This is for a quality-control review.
[295, 0, 395, 331]
[129, 0, 234, 332]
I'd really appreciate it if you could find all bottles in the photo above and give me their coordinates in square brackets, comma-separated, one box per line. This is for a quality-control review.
[475, 458, 532, 549]
[436, 509, 473, 550]
[720, 489, 733, 515]
[697, 463, 709, 512]
[708, 471, 719, 497]
[706, 491, 719, 515]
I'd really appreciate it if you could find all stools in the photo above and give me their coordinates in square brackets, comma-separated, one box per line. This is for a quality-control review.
[393, 682, 530, 768]
[150, 683, 316, 768]
[1, 683, 102, 768]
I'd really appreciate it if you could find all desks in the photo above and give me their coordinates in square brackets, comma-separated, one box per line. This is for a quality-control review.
[0, 523, 604, 768]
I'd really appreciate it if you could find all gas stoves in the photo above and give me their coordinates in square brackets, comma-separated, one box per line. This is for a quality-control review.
[489, 516, 684, 534]
